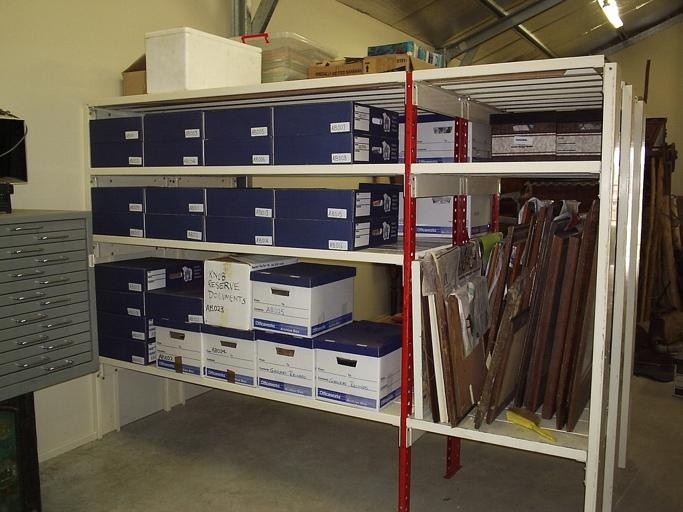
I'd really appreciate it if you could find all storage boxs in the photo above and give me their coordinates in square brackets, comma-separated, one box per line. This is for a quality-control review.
[92, 182, 399, 251]
[314, 321, 402, 412]
[399, 112, 602, 413]
[94, 254, 204, 377]
[251, 262, 355, 339]
[204, 324, 258, 387]
[120, 28, 459, 97]
[254, 329, 315, 400]
[90, 102, 397, 167]
[204, 254, 299, 330]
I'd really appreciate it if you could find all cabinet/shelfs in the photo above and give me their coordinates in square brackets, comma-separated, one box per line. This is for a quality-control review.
[411, 56, 648, 509]
[83, 54, 410, 512]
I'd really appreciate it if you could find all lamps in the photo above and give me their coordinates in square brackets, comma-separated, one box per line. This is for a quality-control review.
[597, 0, 629, 41]
